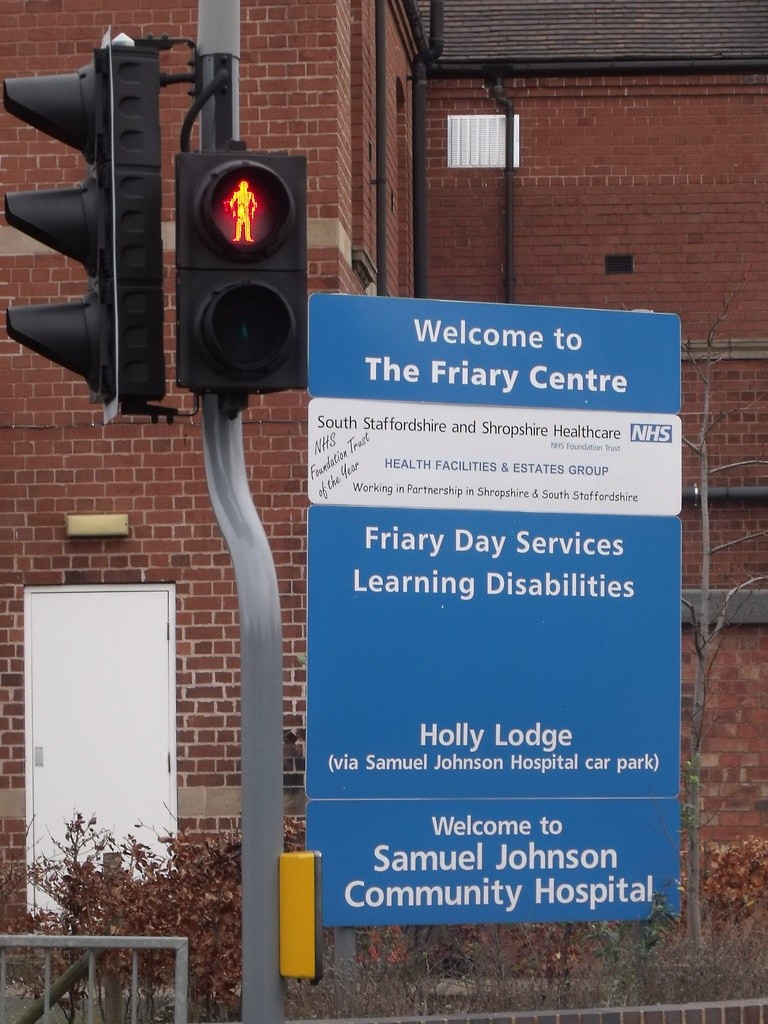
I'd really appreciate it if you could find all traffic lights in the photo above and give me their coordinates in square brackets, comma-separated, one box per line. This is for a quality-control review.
[279, 852, 328, 985]
[2, 24, 168, 427]
[173, 149, 309, 396]
